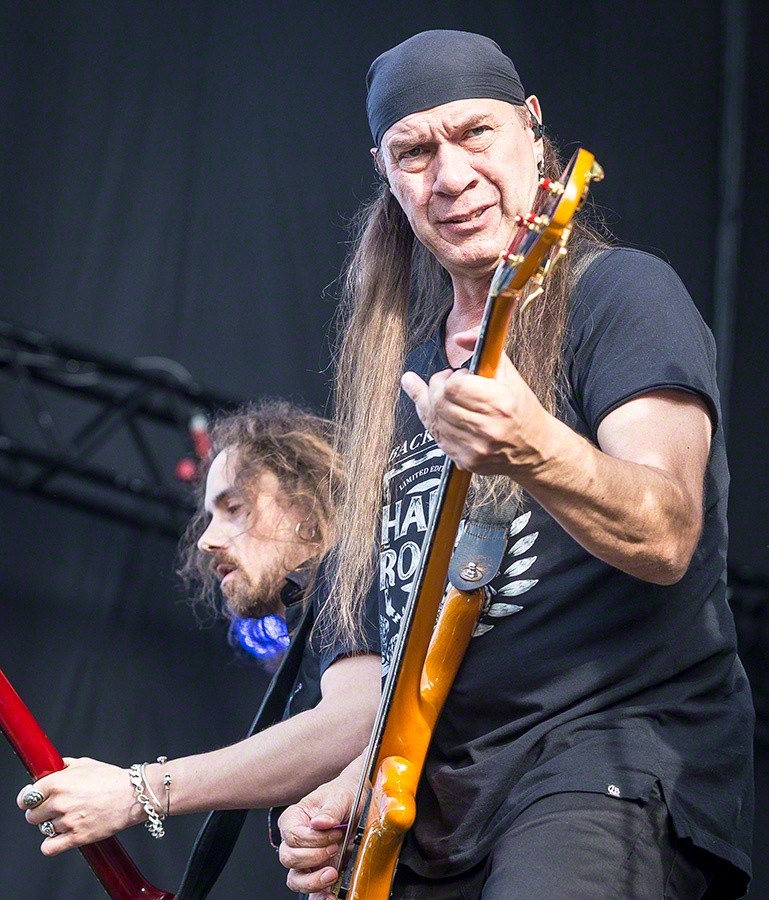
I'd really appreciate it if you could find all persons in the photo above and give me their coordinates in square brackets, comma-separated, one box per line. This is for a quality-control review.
[277, 31, 757, 900]
[17, 400, 384, 857]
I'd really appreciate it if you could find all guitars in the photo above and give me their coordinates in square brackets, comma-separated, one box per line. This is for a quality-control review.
[0, 670, 178, 900]
[324, 146, 604, 900]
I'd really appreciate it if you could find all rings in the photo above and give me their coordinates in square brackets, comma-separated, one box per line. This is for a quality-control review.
[23, 784, 44, 808]
[40, 821, 56, 837]
[423, 419, 430, 431]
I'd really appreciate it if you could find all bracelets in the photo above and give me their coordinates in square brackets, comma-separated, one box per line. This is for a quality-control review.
[130, 756, 171, 840]
